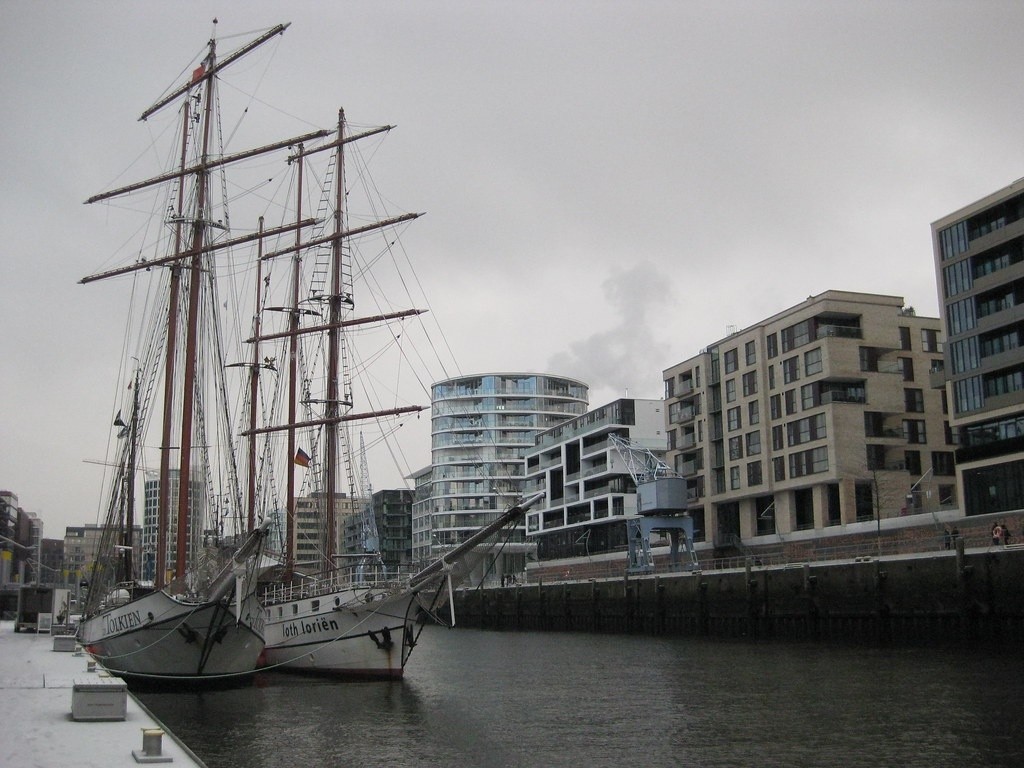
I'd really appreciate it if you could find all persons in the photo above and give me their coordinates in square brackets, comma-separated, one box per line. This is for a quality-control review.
[944, 528, 951, 550]
[991, 521, 1011, 545]
[951, 526, 959, 549]
[500, 573, 505, 587]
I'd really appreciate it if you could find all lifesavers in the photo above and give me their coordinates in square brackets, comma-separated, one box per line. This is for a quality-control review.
[992, 526, 1002, 538]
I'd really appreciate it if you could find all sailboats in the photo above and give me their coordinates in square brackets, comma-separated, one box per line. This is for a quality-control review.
[229, 102, 553, 684]
[73, 14, 343, 682]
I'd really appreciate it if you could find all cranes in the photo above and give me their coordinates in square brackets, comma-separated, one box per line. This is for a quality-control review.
[606, 430, 690, 516]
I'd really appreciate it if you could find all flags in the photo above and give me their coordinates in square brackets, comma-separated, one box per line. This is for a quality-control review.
[294, 448, 311, 468]
